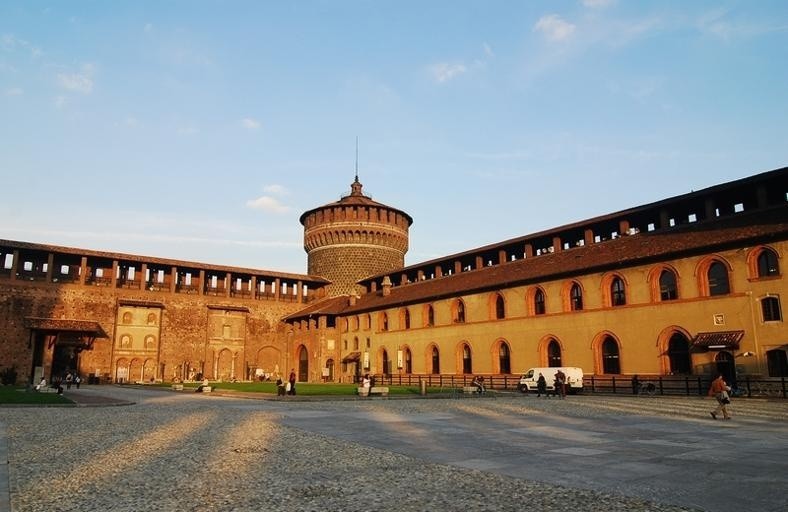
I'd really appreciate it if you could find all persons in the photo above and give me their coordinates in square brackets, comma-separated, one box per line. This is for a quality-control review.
[705, 374, 732, 420]
[33, 376, 47, 391]
[536, 373, 549, 397]
[552, 369, 566, 400]
[363, 374, 371, 388]
[66, 371, 82, 390]
[195, 376, 209, 393]
[631, 374, 638, 395]
[473, 374, 487, 392]
[276, 368, 297, 397]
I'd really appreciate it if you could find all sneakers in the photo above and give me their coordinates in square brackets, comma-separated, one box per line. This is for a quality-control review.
[724, 417, 731, 420]
[710, 412, 717, 420]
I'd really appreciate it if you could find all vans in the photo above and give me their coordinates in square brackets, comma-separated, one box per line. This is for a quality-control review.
[519, 367, 583, 392]
